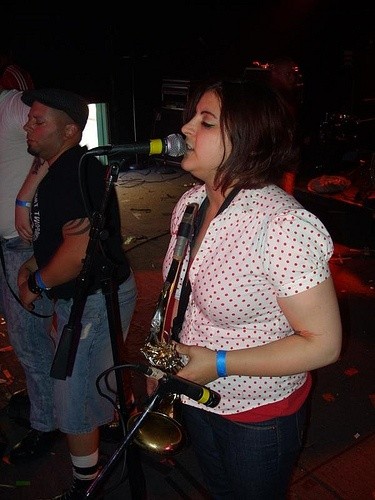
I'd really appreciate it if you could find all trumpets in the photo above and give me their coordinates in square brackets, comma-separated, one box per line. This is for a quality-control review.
[125, 342, 187, 458]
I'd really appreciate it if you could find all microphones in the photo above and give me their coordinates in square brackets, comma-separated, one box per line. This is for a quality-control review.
[137, 362, 221, 408]
[85, 133, 186, 157]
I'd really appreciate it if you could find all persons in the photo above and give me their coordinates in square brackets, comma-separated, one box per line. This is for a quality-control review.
[0, 24, 34, 93]
[17, 89, 137, 500]
[148, 81, 341, 500]
[0, 90, 61, 464]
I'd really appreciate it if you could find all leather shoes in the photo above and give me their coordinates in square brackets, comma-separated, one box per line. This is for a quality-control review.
[7, 405, 31, 428]
[8, 429, 61, 465]
[48, 478, 105, 500]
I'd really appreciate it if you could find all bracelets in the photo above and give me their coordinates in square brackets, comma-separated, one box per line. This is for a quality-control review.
[35, 270, 51, 290]
[16, 199, 31, 208]
[216, 350, 227, 377]
[28, 273, 43, 294]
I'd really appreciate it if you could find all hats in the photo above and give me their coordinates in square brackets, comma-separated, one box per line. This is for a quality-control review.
[20, 88, 89, 131]
